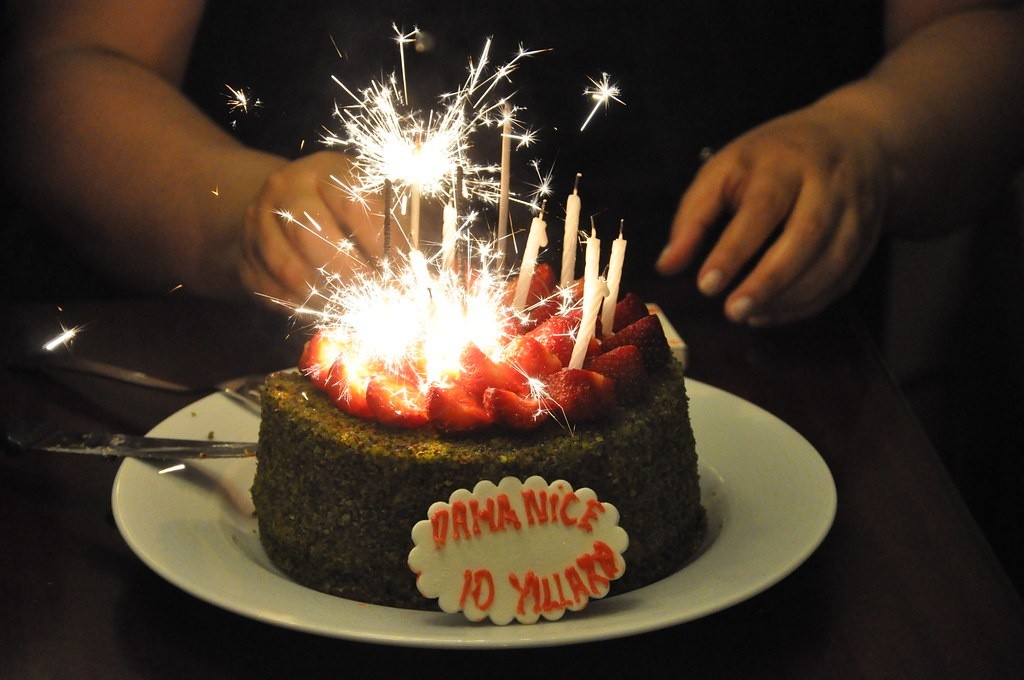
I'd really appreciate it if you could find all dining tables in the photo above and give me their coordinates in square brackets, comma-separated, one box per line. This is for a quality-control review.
[0, 285, 1024, 680]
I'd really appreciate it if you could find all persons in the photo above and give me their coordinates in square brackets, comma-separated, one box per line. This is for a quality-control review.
[13, 0, 1023, 328]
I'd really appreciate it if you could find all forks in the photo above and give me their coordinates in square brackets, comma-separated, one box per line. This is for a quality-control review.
[48, 347, 272, 418]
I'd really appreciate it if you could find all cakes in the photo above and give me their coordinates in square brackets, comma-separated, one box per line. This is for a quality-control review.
[252, 167, 707, 624]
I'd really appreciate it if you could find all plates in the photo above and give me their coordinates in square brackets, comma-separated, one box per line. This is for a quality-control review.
[111, 364, 838, 651]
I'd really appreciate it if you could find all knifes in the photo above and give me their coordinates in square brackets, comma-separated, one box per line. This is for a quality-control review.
[0, 410, 257, 464]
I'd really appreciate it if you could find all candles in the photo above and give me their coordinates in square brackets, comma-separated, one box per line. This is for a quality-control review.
[410, 103, 629, 372]
